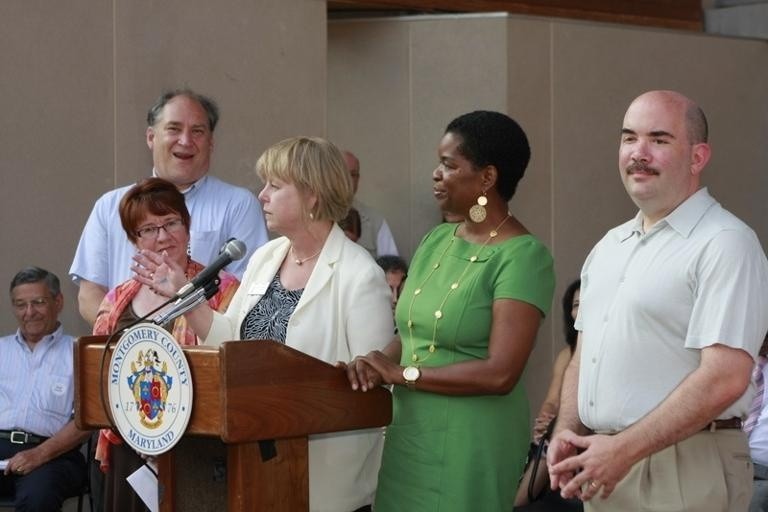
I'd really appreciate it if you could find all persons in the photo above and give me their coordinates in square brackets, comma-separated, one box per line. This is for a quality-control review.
[546, 87, 768, 512]
[514, 278, 578, 505]
[375, 254, 406, 320]
[340, 149, 360, 200]
[70, 89, 270, 327]
[91, 177, 241, 511]
[0, 266, 94, 512]
[340, 208, 362, 243]
[129, 136, 395, 511]
[336, 111, 556, 511]
[742, 333, 768, 512]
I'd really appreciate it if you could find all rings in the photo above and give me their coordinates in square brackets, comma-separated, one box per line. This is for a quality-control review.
[149, 272, 154, 279]
[16, 467, 24, 472]
[590, 480, 599, 488]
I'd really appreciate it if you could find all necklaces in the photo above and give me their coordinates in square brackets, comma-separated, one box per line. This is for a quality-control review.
[406, 212, 514, 363]
[288, 245, 322, 265]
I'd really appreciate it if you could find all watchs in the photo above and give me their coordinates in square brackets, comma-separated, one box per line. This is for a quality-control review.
[402, 364, 422, 391]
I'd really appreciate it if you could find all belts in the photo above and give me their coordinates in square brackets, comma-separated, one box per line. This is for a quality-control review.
[0, 431, 41, 444]
[701, 416, 742, 430]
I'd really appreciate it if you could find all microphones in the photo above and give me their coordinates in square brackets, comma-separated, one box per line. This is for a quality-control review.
[176, 237, 249, 300]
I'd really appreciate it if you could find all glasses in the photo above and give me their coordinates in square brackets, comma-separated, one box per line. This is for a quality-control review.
[14, 296, 48, 310]
[132, 217, 185, 240]
